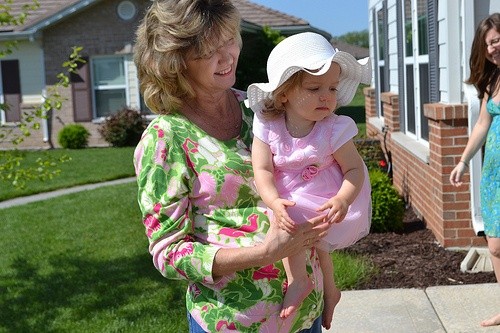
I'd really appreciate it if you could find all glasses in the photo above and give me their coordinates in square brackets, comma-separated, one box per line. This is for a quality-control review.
[486, 36, 500, 48]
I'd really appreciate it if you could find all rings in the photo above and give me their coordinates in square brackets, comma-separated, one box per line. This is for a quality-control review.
[307, 240, 310, 245]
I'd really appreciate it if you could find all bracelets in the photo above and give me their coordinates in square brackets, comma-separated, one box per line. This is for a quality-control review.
[459, 160, 469, 167]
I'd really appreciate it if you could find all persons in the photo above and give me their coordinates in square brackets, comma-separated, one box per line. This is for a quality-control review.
[131, 0, 332, 333]
[450, 13, 500, 326]
[244, 31, 373, 330]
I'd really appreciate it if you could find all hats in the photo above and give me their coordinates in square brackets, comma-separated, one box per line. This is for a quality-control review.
[244, 31, 371, 123]
